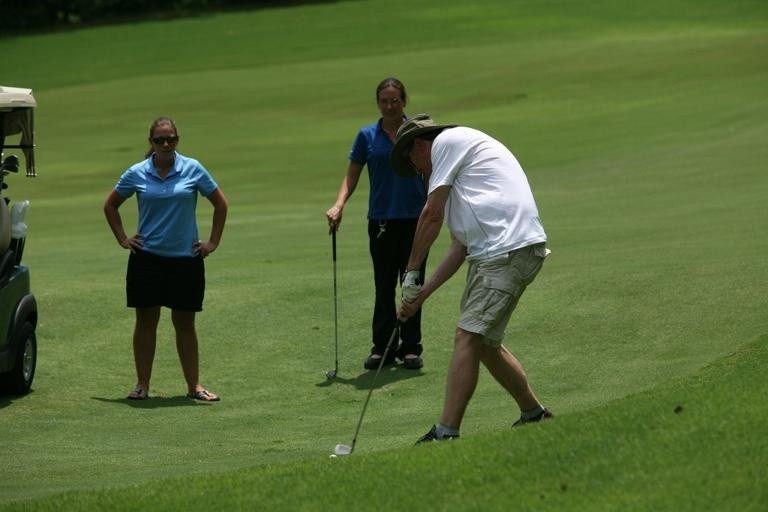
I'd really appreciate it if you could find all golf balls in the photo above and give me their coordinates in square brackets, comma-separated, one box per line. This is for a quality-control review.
[328, 454, 336, 459]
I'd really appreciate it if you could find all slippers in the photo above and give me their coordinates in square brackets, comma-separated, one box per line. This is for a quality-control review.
[128, 387, 148, 400]
[187, 390, 219, 401]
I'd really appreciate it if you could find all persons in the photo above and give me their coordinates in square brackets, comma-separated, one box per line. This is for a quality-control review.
[325, 78, 431, 371]
[391, 114, 555, 449]
[101, 114, 229, 404]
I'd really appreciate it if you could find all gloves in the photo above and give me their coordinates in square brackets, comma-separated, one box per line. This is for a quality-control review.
[402, 267, 424, 303]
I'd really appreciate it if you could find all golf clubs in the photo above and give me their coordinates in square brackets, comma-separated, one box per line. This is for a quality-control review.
[335, 278, 419, 455]
[326, 224, 338, 378]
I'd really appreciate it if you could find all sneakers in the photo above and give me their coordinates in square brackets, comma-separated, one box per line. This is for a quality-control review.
[512, 407, 553, 425]
[416, 424, 459, 442]
[404, 353, 419, 369]
[368, 352, 392, 369]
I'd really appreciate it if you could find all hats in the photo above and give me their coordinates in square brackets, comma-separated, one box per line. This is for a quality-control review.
[390, 113, 459, 179]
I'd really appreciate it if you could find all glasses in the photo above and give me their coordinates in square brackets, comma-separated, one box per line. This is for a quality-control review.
[152, 135, 178, 144]
